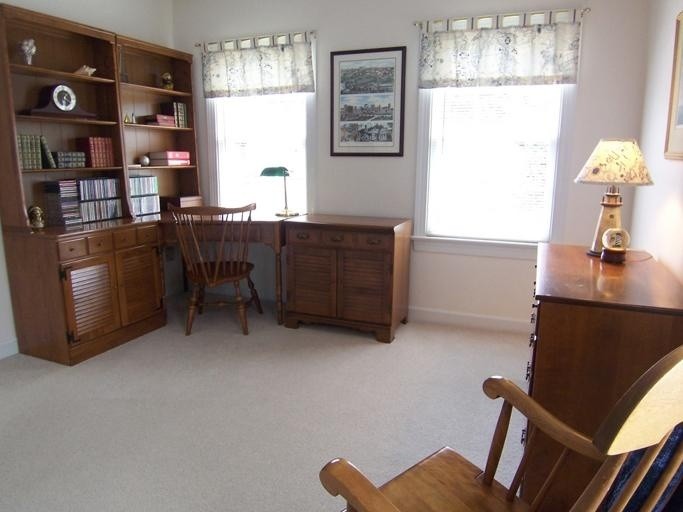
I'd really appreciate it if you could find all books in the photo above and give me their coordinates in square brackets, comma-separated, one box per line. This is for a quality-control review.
[14, 132, 202, 227]
[138, 100, 189, 128]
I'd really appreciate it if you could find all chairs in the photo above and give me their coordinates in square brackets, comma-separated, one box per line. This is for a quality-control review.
[319, 343, 683, 512]
[165, 203, 263, 335]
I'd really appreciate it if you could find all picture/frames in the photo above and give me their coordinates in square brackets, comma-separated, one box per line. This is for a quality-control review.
[329, 46, 406, 157]
[661, 11, 683, 159]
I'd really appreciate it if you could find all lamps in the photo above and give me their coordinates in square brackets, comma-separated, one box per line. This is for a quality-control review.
[259, 166, 298, 217]
[572, 139, 653, 258]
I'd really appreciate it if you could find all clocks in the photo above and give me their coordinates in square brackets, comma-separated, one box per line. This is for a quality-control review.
[48, 82, 77, 116]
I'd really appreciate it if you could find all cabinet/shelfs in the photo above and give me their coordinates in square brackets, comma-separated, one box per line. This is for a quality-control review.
[283, 220, 408, 343]
[518, 242, 683, 512]
[160, 210, 283, 325]
